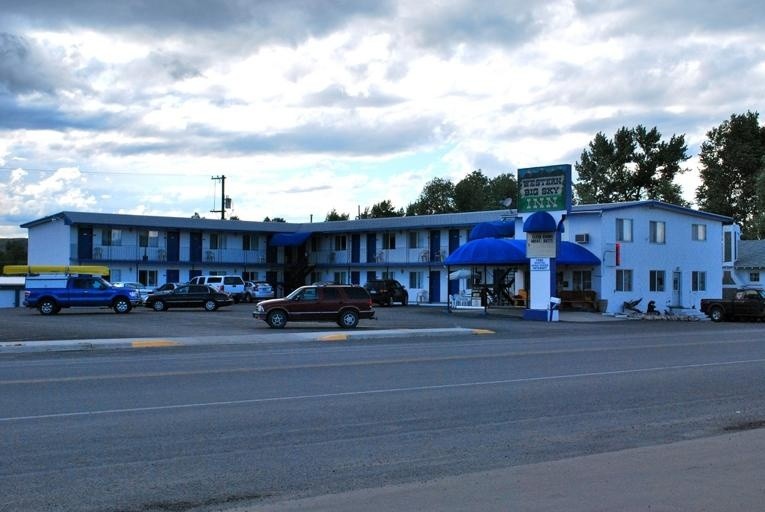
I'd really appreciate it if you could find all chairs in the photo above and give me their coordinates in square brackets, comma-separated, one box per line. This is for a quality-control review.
[92, 247, 103, 260]
[206, 250, 215, 261]
[157, 249, 167, 261]
[418, 249, 429, 262]
[449, 294, 469, 309]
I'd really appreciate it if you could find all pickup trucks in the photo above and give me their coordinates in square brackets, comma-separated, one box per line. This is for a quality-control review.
[21, 273, 143, 315]
[700, 286, 765, 324]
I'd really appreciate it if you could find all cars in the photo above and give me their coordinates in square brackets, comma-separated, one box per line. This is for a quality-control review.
[243, 281, 275, 303]
[154, 282, 188, 293]
[112, 281, 154, 307]
[143, 283, 234, 311]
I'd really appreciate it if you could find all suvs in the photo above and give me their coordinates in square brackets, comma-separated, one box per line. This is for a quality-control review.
[185, 275, 246, 305]
[362, 279, 408, 308]
[250, 280, 376, 330]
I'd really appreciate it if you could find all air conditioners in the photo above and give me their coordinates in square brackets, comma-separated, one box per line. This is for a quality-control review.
[575, 233, 590, 243]
